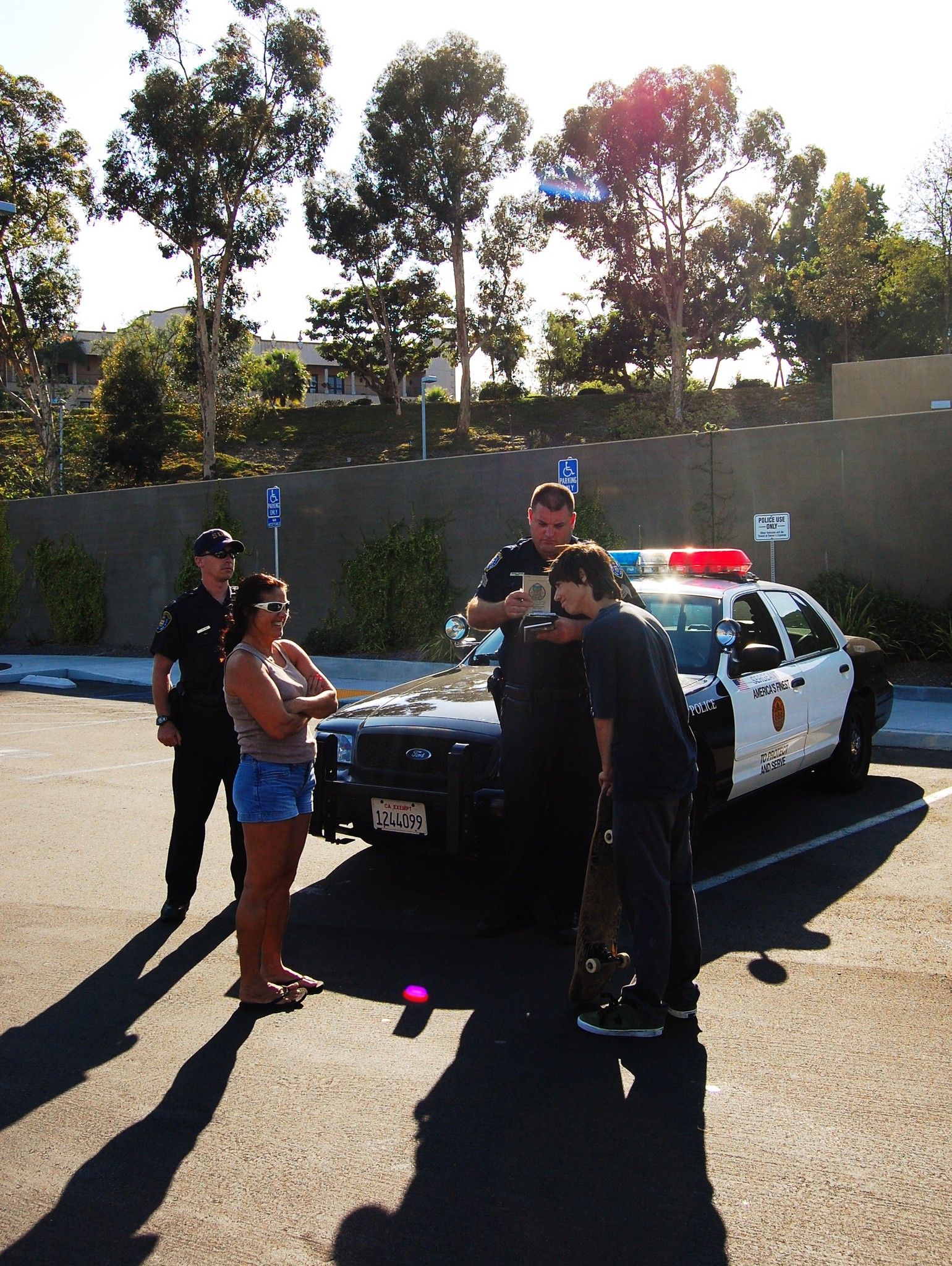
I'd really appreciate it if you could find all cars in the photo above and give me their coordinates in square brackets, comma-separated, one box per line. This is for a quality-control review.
[303, 545, 898, 867]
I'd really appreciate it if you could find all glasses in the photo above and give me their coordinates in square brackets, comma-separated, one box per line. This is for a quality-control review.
[251, 601, 291, 613]
[201, 548, 240, 559]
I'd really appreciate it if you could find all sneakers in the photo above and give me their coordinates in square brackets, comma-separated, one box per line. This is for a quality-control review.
[577, 991, 664, 1037]
[662, 998, 697, 1020]
[161, 899, 189, 922]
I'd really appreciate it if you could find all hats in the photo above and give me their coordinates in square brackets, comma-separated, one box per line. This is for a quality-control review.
[194, 528, 245, 556]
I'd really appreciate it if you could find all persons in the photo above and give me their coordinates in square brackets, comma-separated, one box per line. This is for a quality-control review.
[217, 572, 339, 1007]
[149, 529, 247, 922]
[543, 539, 702, 1038]
[466, 483, 647, 944]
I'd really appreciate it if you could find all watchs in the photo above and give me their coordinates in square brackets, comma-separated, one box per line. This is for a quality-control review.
[156, 715, 172, 726]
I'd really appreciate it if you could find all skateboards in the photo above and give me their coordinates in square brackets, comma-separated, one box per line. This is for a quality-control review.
[569, 783, 630, 1004]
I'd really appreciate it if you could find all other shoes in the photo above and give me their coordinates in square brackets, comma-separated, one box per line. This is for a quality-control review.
[476, 912, 540, 938]
[557, 910, 577, 944]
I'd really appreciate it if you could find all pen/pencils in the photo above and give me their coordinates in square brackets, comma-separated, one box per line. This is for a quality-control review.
[520, 588, 523, 602]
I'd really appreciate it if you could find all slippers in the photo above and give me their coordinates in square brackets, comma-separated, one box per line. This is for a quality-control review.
[268, 975, 325, 991]
[240, 986, 307, 1010]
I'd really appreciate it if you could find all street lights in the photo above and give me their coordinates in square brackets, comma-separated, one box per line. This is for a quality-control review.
[421, 376, 440, 461]
[51, 397, 67, 495]
